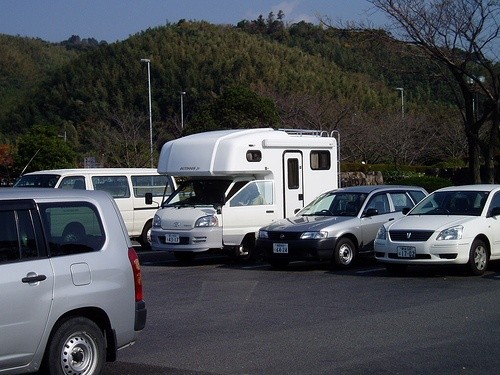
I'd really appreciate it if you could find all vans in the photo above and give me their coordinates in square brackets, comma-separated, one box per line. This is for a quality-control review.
[13, 169, 181, 250]
[145, 126, 338, 264]
[0, 188, 147, 375]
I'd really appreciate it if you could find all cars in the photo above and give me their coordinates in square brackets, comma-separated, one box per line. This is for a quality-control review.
[257, 184, 438, 270]
[373, 184, 500, 276]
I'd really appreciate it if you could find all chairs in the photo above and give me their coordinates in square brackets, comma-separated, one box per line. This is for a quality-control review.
[452, 197, 485, 215]
[53, 221, 86, 253]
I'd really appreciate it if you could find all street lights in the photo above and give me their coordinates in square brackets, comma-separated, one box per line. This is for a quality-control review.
[140, 58, 153, 168]
[396, 88, 405, 120]
[180, 92, 186, 128]
[467, 75, 485, 117]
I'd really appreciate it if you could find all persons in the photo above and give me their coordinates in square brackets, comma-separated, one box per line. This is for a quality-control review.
[241, 185, 268, 205]
